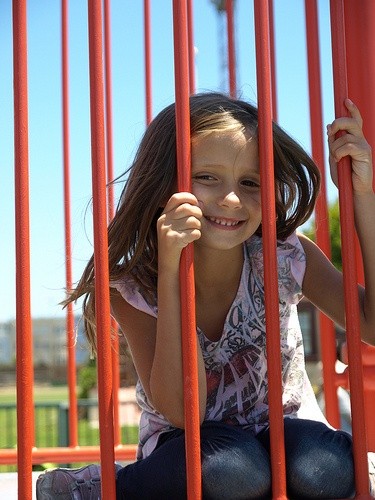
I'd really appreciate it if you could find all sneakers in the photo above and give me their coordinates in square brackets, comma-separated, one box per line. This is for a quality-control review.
[36, 462, 123, 500]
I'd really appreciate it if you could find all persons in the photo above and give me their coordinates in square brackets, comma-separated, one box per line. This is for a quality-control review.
[36, 91, 375, 500]
